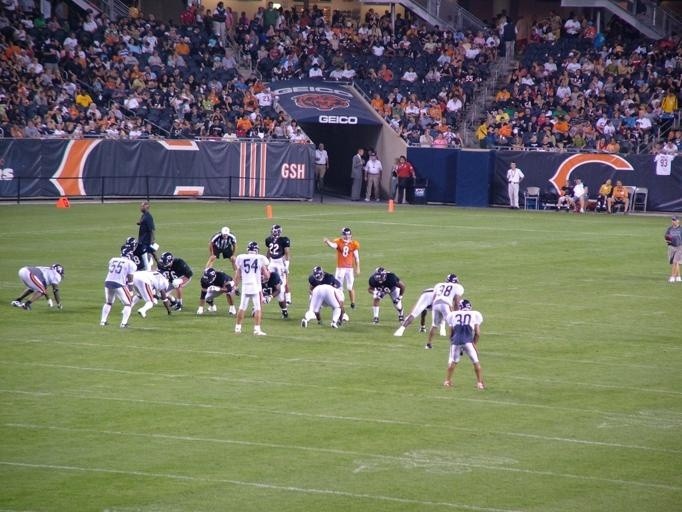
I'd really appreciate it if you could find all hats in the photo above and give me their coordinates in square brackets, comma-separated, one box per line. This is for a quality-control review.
[372, 152, 375, 156]
[221, 227, 229, 234]
[672, 215, 679, 220]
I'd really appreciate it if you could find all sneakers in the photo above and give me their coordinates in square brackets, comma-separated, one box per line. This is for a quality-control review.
[393, 326, 405, 336]
[440, 320, 446, 337]
[460, 348, 463, 355]
[206, 306, 266, 336]
[170, 297, 177, 308]
[342, 313, 349, 321]
[175, 302, 181, 311]
[331, 323, 338, 328]
[364, 198, 370, 202]
[286, 301, 292, 306]
[676, 276, 682, 282]
[603, 205, 607, 209]
[580, 207, 585, 214]
[301, 318, 307, 328]
[372, 316, 404, 325]
[22, 303, 32, 311]
[11, 301, 23, 307]
[99, 309, 146, 328]
[556, 208, 559, 211]
[425, 343, 432, 349]
[282, 310, 288, 320]
[566, 209, 569, 212]
[668, 276, 675, 283]
[351, 303, 355, 308]
[444, 380, 487, 390]
[596, 206, 600, 209]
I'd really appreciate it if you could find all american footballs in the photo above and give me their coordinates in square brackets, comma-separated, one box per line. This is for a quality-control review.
[665, 233, 672, 241]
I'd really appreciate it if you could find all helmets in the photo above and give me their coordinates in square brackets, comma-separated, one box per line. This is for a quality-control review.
[313, 265, 324, 282]
[446, 274, 458, 283]
[374, 267, 387, 283]
[158, 252, 174, 268]
[126, 237, 138, 251]
[204, 268, 216, 284]
[51, 264, 63, 274]
[458, 299, 472, 311]
[341, 227, 352, 242]
[121, 244, 133, 257]
[261, 269, 270, 283]
[271, 225, 282, 239]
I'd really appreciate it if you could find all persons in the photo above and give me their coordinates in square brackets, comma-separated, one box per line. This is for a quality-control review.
[424, 274, 465, 350]
[1, 0, 682, 158]
[349, 144, 382, 205]
[393, 288, 447, 336]
[300, 284, 345, 329]
[395, 156, 416, 205]
[442, 298, 486, 391]
[323, 227, 361, 311]
[664, 216, 682, 284]
[368, 267, 406, 325]
[389, 157, 400, 200]
[307, 265, 345, 324]
[99, 199, 292, 337]
[505, 161, 525, 210]
[554, 177, 631, 214]
[315, 143, 329, 194]
[10, 264, 65, 310]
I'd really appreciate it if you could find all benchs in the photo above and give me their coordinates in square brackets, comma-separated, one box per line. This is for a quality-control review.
[542, 202, 623, 213]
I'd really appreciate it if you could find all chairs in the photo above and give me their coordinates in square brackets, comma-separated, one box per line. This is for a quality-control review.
[633, 187, 649, 212]
[623, 185, 636, 211]
[524, 187, 541, 210]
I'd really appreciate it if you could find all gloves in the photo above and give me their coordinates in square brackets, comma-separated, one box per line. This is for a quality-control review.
[393, 298, 400, 304]
[172, 279, 183, 289]
[57, 304, 62, 310]
[207, 285, 221, 293]
[284, 269, 288, 273]
[420, 328, 426, 333]
[339, 323, 344, 328]
[235, 289, 241, 296]
[265, 295, 273, 304]
[196, 306, 203, 314]
[48, 298, 53, 307]
[377, 291, 384, 299]
[317, 320, 322, 324]
